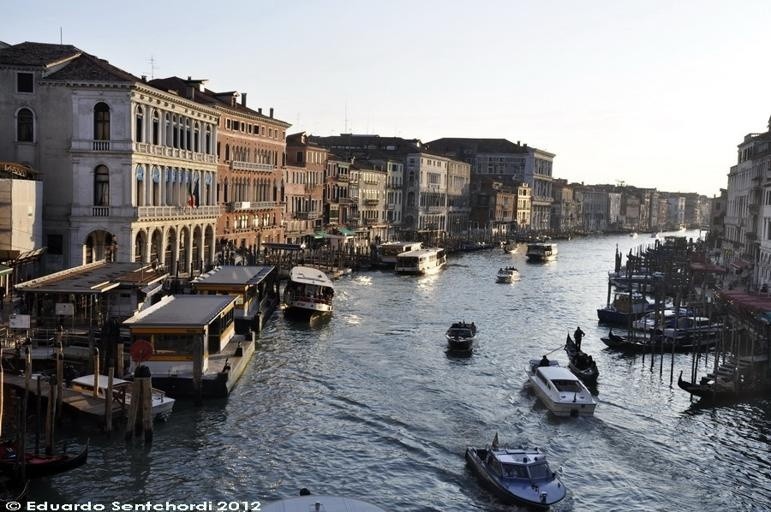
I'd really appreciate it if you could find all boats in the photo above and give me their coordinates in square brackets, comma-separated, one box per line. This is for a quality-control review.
[601, 228, 771, 406]
[395, 248, 448, 274]
[66, 373, 176, 421]
[563, 332, 600, 380]
[463, 433, 568, 506]
[445, 321, 480, 349]
[0, 436, 91, 482]
[527, 243, 558, 261]
[497, 269, 521, 281]
[503, 240, 520, 253]
[284, 267, 334, 318]
[524, 356, 596, 419]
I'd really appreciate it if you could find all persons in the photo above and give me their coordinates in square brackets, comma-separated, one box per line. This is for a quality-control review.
[539, 355, 550, 366]
[609, 327, 626, 342]
[574, 326, 585, 352]
